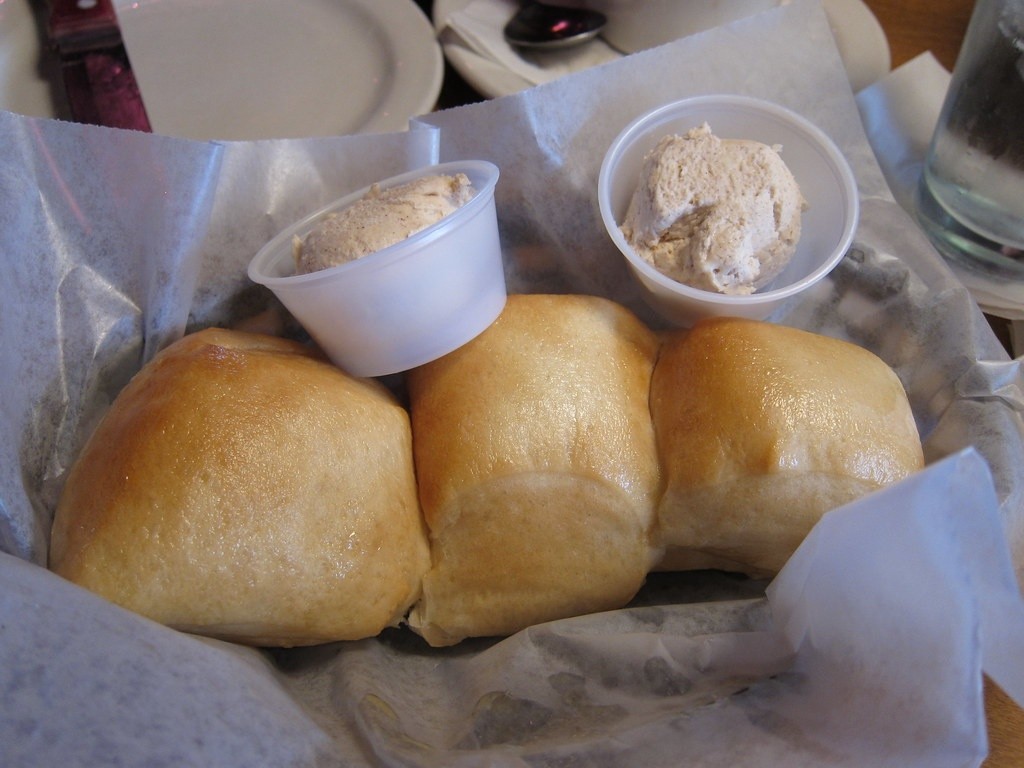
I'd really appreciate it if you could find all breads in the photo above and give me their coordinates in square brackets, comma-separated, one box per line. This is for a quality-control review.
[50, 328, 428, 647]
[410, 295, 663, 648]
[650, 317, 925, 577]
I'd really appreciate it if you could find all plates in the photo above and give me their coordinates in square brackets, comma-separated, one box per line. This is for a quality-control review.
[0, 0, 444, 133]
[434, 0, 891, 99]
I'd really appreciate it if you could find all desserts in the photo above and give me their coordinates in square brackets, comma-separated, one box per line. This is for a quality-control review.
[620, 117, 805, 297]
[289, 171, 475, 278]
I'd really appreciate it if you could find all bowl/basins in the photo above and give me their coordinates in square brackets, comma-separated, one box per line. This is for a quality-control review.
[246, 160, 507, 379]
[597, 96, 859, 331]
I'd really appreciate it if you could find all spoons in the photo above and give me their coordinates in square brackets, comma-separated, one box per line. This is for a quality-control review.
[504, 0, 608, 50]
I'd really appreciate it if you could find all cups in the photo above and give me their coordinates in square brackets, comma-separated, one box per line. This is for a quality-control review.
[914, 0, 1024, 283]
